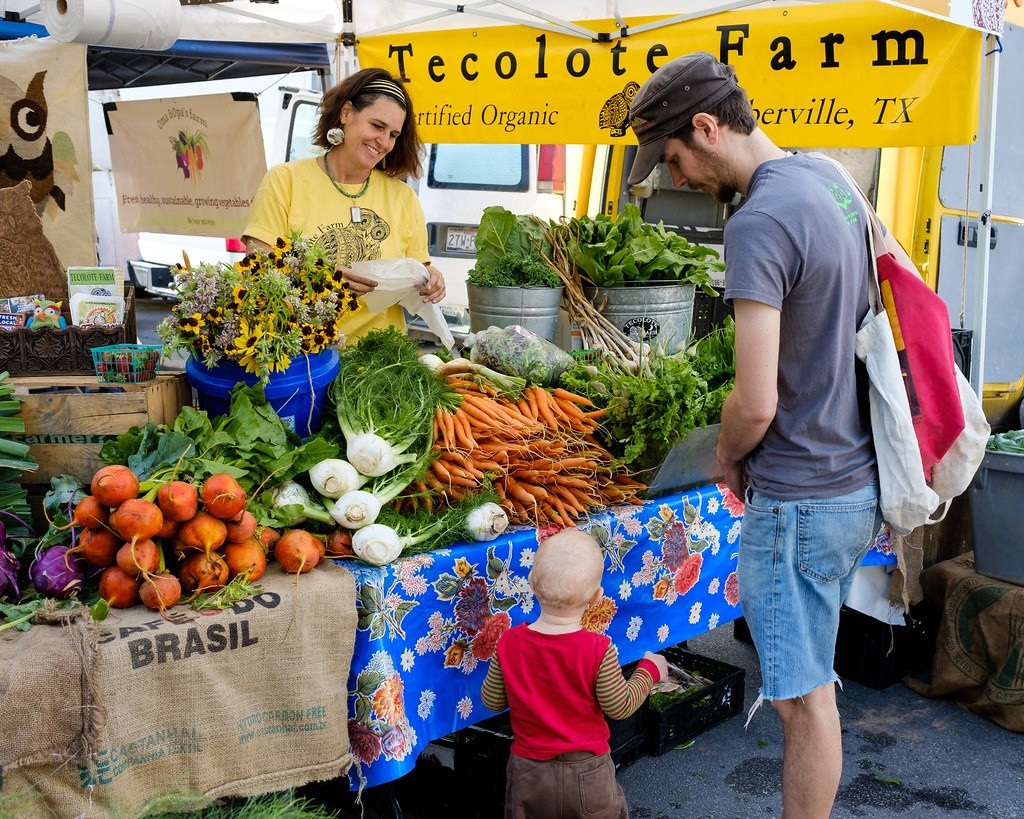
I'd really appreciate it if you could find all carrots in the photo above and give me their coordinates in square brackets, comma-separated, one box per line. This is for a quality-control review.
[396, 378, 648, 527]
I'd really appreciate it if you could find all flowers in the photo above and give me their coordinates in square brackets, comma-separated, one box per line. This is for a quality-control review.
[156, 232, 358, 385]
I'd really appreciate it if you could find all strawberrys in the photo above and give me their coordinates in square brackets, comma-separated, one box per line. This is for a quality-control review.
[97, 347, 158, 382]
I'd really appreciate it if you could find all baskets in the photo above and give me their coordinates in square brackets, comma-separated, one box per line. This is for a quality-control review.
[91, 344, 163, 385]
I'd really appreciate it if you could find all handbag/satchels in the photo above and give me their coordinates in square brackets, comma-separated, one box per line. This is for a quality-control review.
[807, 152, 991, 536]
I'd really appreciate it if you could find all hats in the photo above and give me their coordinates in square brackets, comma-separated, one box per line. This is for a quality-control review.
[627, 52, 738, 185]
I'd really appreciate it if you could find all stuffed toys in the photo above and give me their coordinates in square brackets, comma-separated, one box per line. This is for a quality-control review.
[29, 298, 63, 333]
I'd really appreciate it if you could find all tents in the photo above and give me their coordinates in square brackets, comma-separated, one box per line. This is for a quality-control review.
[1, 0, 1004, 449]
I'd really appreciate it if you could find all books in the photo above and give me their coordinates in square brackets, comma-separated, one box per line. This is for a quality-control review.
[66, 263, 126, 329]
[0, 295, 46, 332]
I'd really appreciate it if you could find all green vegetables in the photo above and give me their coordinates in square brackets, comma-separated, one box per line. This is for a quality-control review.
[472, 317, 736, 470]
[101, 329, 500, 564]
[467, 206, 726, 295]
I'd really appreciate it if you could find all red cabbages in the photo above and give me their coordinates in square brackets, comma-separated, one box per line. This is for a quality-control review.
[0, 548, 21, 595]
[33, 545, 79, 595]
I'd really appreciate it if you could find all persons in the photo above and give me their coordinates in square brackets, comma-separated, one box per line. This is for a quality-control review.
[627, 51, 884, 819]
[238, 65, 446, 363]
[481, 530, 668, 819]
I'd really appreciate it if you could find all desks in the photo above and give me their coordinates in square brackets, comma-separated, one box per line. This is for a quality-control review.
[0, 482, 923, 819]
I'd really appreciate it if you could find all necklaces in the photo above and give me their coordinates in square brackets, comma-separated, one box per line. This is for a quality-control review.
[321, 149, 371, 224]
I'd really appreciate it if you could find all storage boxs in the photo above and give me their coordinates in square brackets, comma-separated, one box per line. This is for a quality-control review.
[0, 371, 184, 482]
[91, 343, 163, 382]
[464, 718, 647, 775]
[624, 648, 744, 756]
[970, 430, 1024, 586]
[0, 285, 138, 377]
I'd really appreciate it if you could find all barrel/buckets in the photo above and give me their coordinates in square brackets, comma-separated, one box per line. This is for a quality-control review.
[187, 346, 340, 437]
[590, 283, 696, 356]
[465, 280, 564, 343]
